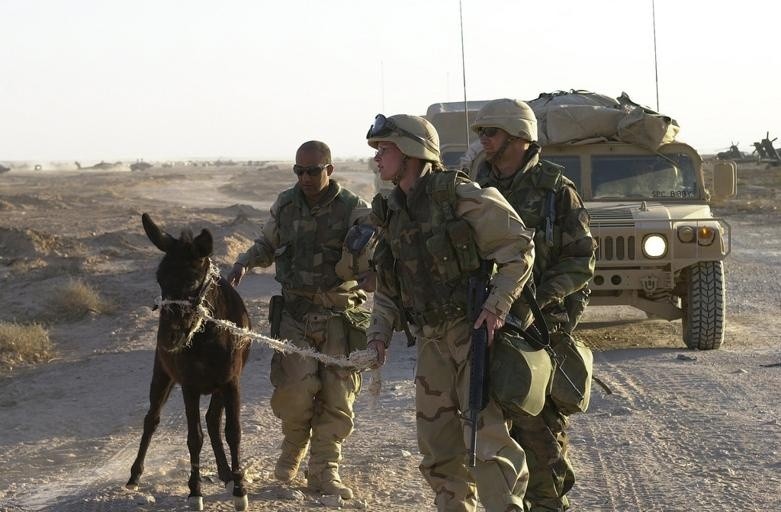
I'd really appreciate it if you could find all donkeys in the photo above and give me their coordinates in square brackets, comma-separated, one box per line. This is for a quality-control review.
[125, 210, 253, 512]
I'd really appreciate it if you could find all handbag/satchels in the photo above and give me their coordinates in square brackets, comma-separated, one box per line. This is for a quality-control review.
[342, 306, 373, 358]
[488, 329, 554, 419]
[549, 333, 595, 415]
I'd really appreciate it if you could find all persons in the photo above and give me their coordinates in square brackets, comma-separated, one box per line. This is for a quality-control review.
[225, 139, 375, 499]
[470, 98, 601, 511]
[364, 113, 538, 512]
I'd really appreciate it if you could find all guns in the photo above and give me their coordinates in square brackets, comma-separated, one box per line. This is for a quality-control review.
[465, 260, 495, 467]
[268, 295, 285, 340]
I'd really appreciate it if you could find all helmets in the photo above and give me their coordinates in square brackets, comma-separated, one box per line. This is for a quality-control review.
[470, 98, 539, 143]
[367, 114, 442, 163]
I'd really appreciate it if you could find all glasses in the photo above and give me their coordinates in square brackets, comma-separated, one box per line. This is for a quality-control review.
[477, 126, 499, 137]
[293, 163, 331, 176]
[366, 113, 389, 137]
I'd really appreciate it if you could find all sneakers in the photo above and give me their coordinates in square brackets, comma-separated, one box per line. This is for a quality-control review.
[306, 463, 354, 499]
[274, 439, 311, 483]
